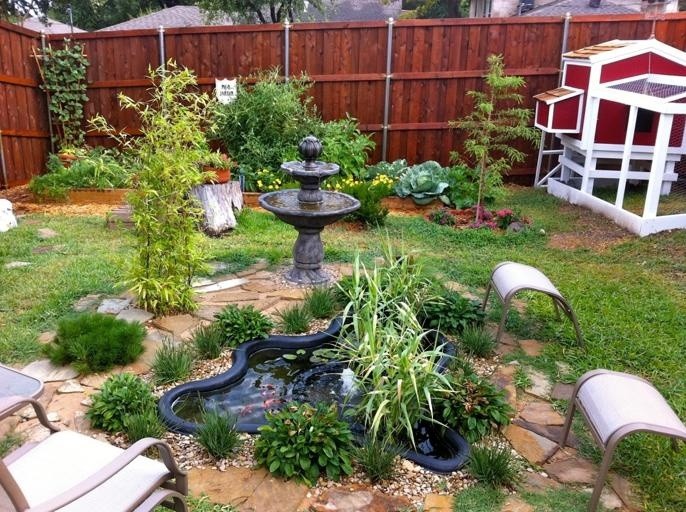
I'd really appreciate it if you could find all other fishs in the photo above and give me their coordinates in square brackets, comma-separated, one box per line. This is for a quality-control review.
[209, 383, 285, 422]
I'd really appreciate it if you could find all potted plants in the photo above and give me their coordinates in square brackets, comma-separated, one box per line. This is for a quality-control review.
[202, 152, 234, 183]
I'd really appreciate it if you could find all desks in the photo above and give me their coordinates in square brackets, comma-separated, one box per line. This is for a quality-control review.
[1, 366, 45, 420]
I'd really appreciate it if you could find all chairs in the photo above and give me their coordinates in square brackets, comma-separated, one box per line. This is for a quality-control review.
[0, 399, 188, 512]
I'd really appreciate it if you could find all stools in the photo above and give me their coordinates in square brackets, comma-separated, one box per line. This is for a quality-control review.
[558, 368, 686, 512]
[480, 261, 584, 351]
